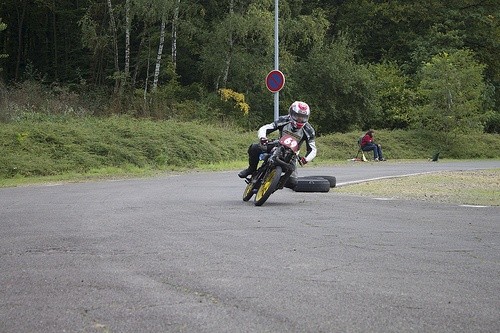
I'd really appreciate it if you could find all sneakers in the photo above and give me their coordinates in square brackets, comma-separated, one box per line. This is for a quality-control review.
[374, 158, 379, 161]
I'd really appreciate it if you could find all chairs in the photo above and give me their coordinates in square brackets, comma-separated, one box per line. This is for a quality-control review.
[356, 136, 371, 161]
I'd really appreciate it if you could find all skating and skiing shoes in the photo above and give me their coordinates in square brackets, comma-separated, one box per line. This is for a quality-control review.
[380, 158, 386, 161]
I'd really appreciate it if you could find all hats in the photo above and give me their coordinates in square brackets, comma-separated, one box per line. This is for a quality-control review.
[368, 129, 375, 133]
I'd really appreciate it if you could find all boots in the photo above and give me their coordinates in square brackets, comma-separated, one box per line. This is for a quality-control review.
[238, 150, 258, 178]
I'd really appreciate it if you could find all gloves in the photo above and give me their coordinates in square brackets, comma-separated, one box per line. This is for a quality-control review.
[260, 137, 268, 146]
[299, 157, 307, 166]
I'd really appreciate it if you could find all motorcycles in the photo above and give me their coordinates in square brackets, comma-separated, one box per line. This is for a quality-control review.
[242, 134, 302, 206]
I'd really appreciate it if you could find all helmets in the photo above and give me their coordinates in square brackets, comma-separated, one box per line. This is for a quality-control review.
[289, 101, 310, 129]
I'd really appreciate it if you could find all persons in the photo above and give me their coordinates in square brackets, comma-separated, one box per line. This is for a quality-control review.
[238, 100, 317, 188]
[360, 129, 387, 162]
